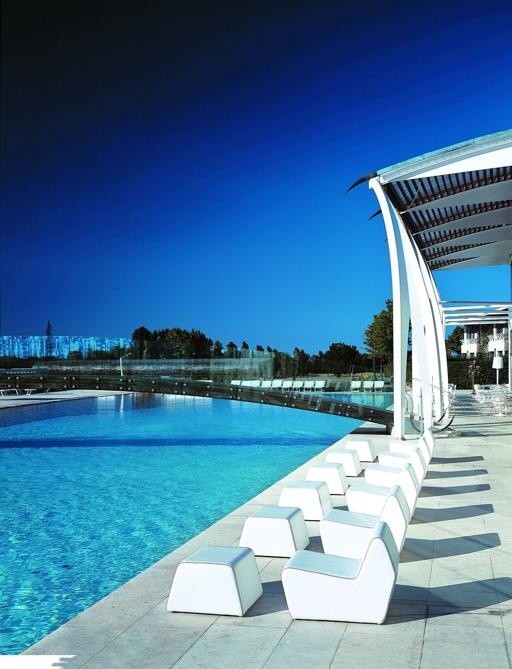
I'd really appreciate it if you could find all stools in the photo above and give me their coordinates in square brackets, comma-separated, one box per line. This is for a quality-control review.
[324, 448, 364, 478]
[167, 545, 264, 618]
[239, 505, 310, 559]
[345, 439, 378, 463]
[277, 479, 334, 522]
[306, 462, 348, 497]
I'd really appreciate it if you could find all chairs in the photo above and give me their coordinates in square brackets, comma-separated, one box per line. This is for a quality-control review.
[282, 522, 401, 625]
[319, 486, 412, 560]
[388, 427, 435, 458]
[364, 447, 427, 484]
[345, 463, 421, 523]
